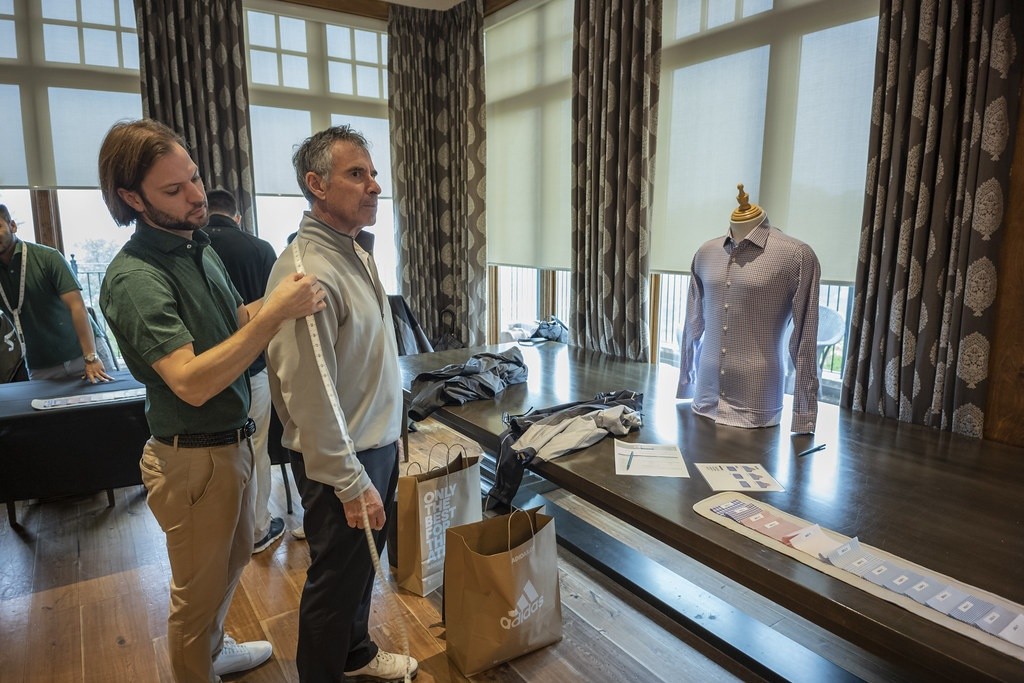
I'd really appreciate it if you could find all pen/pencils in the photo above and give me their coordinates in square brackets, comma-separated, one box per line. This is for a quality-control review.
[626, 450, 634, 470]
[797, 442, 826, 457]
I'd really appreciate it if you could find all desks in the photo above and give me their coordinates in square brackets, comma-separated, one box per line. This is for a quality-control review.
[1, 368, 293, 534]
[398, 337, 1023, 682]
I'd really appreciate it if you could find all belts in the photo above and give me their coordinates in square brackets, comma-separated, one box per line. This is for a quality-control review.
[153, 416, 257, 448]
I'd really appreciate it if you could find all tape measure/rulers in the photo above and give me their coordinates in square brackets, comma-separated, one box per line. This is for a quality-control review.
[289, 234, 412, 682]
[0, 241, 28, 357]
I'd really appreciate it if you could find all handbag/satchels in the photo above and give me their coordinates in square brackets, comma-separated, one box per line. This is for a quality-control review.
[445, 494, 563, 678]
[398, 442, 481, 600]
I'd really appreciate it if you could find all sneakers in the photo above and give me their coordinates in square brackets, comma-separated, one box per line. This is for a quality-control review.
[211, 635, 271, 674]
[251, 517, 285, 552]
[344, 642, 419, 682]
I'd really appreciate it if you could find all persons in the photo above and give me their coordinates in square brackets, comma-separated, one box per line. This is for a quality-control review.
[98, 117, 326, 682]
[0, 204, 116, 388]
[261, 124, 419, 681]
[197, 190, 286, 555]
[674, 182, 822, 434]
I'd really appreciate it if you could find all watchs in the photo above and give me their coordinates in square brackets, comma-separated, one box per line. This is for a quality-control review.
[84, 353, 99, 363]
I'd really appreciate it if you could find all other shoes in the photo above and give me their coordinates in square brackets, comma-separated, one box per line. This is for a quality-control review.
[291, 527, 307, 539]
